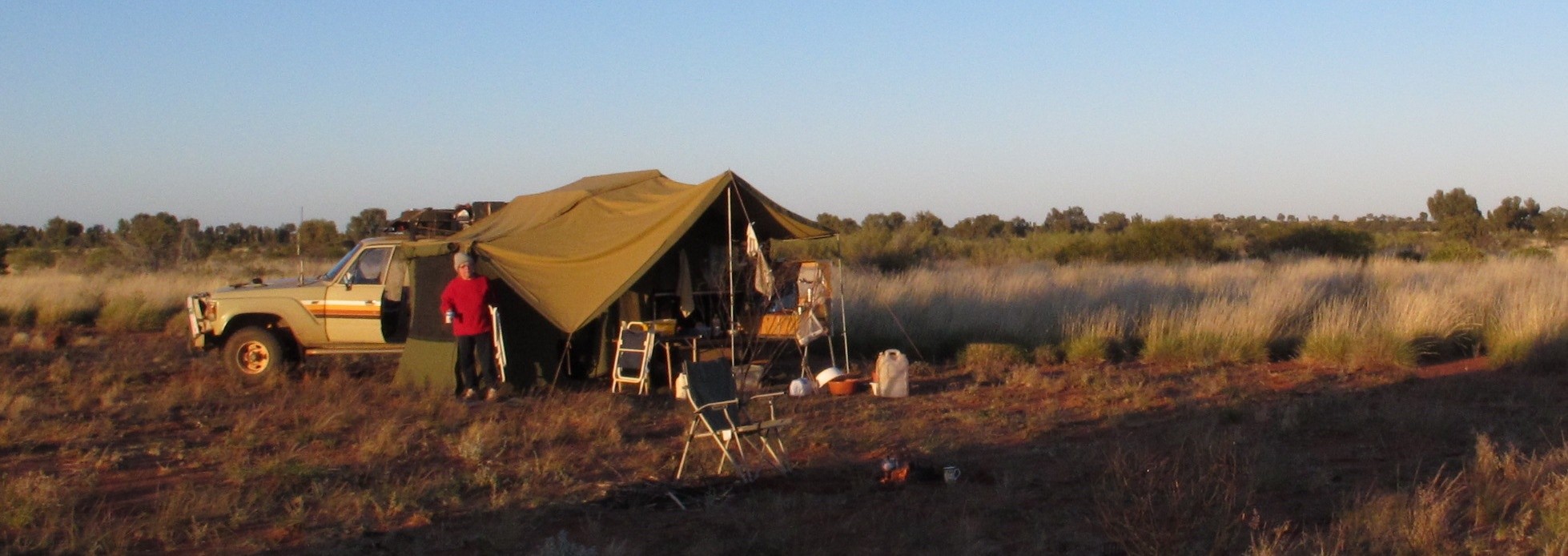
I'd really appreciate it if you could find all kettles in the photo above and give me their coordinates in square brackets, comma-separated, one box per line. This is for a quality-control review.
[880, 455, 899, 472]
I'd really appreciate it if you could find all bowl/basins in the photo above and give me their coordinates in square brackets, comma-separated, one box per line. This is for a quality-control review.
[870, 382, 878, 396]
[816, 367, 845, 389]
[828, 378, 858, 395]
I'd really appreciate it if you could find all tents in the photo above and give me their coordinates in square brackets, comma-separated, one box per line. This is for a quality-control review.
[390, 168, 849, 390]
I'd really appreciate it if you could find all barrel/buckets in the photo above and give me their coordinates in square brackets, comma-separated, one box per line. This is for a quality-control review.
[876, 349, 908, 398]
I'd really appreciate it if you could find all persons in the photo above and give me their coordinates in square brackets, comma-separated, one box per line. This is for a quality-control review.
[440, 252, 500, 400]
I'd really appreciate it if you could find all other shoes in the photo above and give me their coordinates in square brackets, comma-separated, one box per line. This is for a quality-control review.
[464, 388, 476, 400]
[486, 387, 497, 401]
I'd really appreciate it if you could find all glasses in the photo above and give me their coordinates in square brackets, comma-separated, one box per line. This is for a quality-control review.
[459, 265, 468, 270]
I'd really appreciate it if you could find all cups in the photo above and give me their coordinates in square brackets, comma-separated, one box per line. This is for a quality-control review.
[942, 467, 961, 483]
[446, 311, 454, 324]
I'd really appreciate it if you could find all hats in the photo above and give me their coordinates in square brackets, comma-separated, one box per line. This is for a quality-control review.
[453, 252, 474, 272]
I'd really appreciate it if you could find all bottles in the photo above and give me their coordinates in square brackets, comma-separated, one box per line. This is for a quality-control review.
[675, 373, 687, 399]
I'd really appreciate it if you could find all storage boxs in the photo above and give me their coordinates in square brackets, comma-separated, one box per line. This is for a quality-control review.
[472, 201, 508, 223]
[737, 311, 799, 335]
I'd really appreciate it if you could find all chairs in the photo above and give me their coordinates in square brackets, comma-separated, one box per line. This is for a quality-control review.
[381, 256, 406, 330]
[611, 320, 655, 397]
[674, 355, 793, 488]
[474, 304, 507, 385]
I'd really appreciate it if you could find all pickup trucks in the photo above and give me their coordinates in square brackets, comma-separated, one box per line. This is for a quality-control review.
[186, 233, 748, 386]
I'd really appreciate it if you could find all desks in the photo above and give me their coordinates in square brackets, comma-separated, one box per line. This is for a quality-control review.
[654, 333, 704, 391]
[758, 328, 837, 396]
